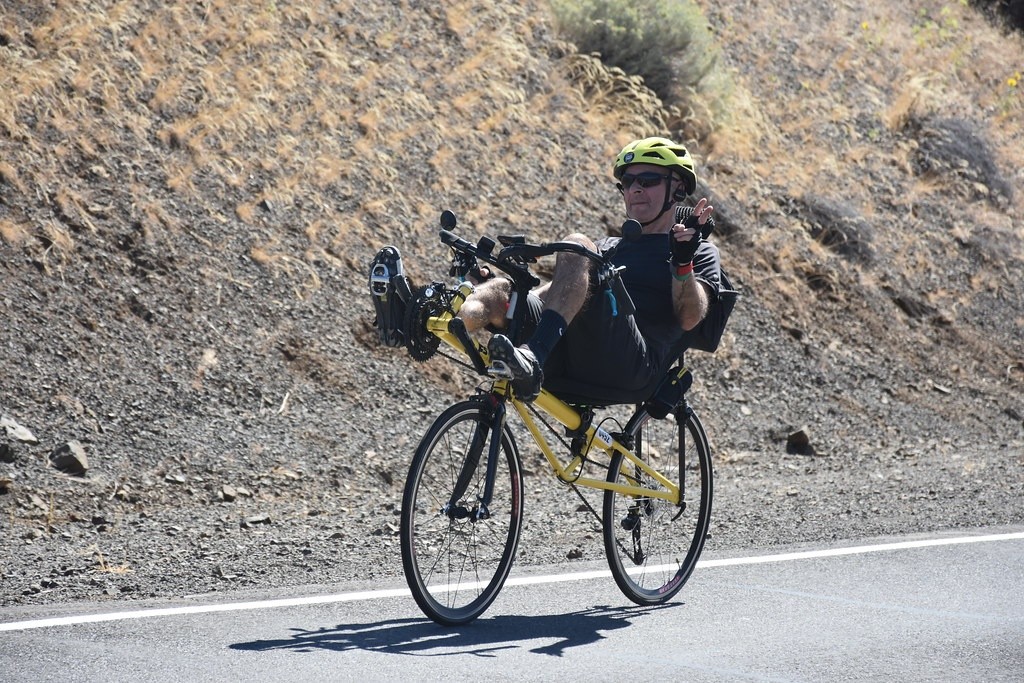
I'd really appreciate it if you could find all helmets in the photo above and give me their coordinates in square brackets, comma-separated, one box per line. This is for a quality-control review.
[612, 136, 698, 195]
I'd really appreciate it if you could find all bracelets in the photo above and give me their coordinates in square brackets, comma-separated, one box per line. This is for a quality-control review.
[669, 255, 694, 282]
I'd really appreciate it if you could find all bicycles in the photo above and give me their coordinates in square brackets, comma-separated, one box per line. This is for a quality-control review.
[371, 210, 714, 626]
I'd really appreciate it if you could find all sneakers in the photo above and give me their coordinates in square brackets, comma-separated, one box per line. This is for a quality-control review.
[488, 332, 545, 404]
[366, 244, 416, 348]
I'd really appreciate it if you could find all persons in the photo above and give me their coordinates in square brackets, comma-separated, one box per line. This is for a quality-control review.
[369, 137, 723, 408]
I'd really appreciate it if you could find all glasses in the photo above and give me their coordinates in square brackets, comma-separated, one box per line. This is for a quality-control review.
[621, 171, 680, 189]
